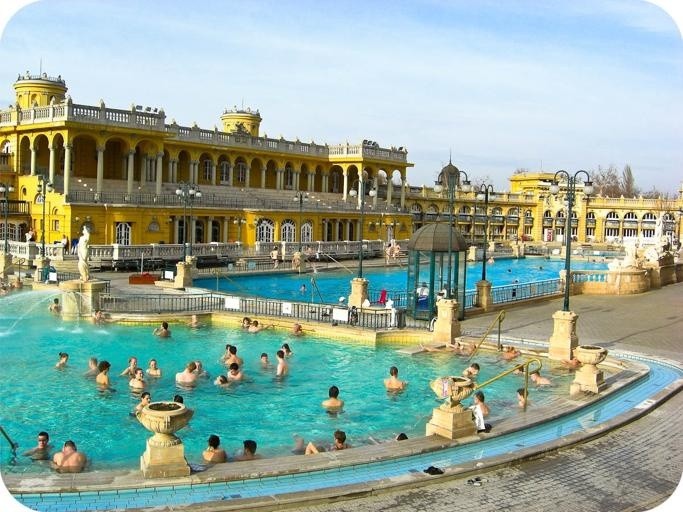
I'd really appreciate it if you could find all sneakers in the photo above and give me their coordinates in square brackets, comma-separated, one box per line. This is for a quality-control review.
[430, 468, 443, 474]
[424, 467, 434, 473]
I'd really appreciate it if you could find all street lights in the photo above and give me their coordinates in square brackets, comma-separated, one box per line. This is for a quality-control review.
[549, 170, 595, 311]
[434, 170, 471, 299]
[476, 184, 496, 280]
[175, 182, 203, 256]
[37, 175, 53, 256]
[349, 179, 377, 277]
[292, 191, 309, 251]
[0, 180, 15, 253]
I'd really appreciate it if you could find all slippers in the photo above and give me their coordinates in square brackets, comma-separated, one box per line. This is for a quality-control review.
[463, 480, 481, 487]
[472, 477, 487, 483]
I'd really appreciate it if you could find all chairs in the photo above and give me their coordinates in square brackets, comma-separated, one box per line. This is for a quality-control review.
[377, 290, 386, 307]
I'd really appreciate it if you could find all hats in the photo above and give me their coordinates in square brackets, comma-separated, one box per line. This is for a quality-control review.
[339, 297, 345, 301]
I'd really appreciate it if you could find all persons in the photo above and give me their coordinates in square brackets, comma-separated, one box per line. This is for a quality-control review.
[153, 322, 168, 337]
[323, 386, 343, 406]
[468, 392, 489, 419]
[319, 427, 355, 453]
[91, 308, 120, 323]
[83, 344, 292, 410]
[269, 245, 312, 268]
[242, 317, 273, 333]
[371, 430, 410, 446]
[514, 364, 552, 407]
[56, 352, 68, 368]
[203, 434, 227, 462]
[233, 440, 262, 462]
[488, 256, 494, 263]
[50, 440, 90, 473]
[417, 282, 447, 298]
[35, 430, 90, 473]
[305, 431, 355, 456]
[78, 225, 91, 281]
[51, 298, 62, 311]
[293, 324, 301, 333]
[463, 363, 480, 376]
[202, 434, 262, 462]
[292, 433, 324, 455]
[29, 227, 35, 241]
[22, 431, 54, 460]
[384, 366, 407, 389]
[61, 234, 68, 244]
[385, 240, 400, 266]
